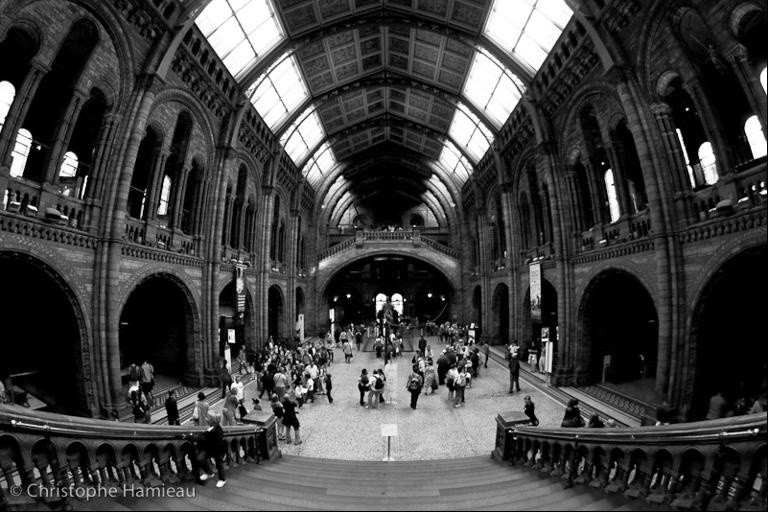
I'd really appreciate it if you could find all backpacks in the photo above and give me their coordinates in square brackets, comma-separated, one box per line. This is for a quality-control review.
[374, 376, 384, 389]
[407, 374, 420, 392]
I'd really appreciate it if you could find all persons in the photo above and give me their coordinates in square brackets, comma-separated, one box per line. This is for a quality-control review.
[183, 410, 228, 489]
[367, 222, 405, 231]
[126, 317, 765, 444]
